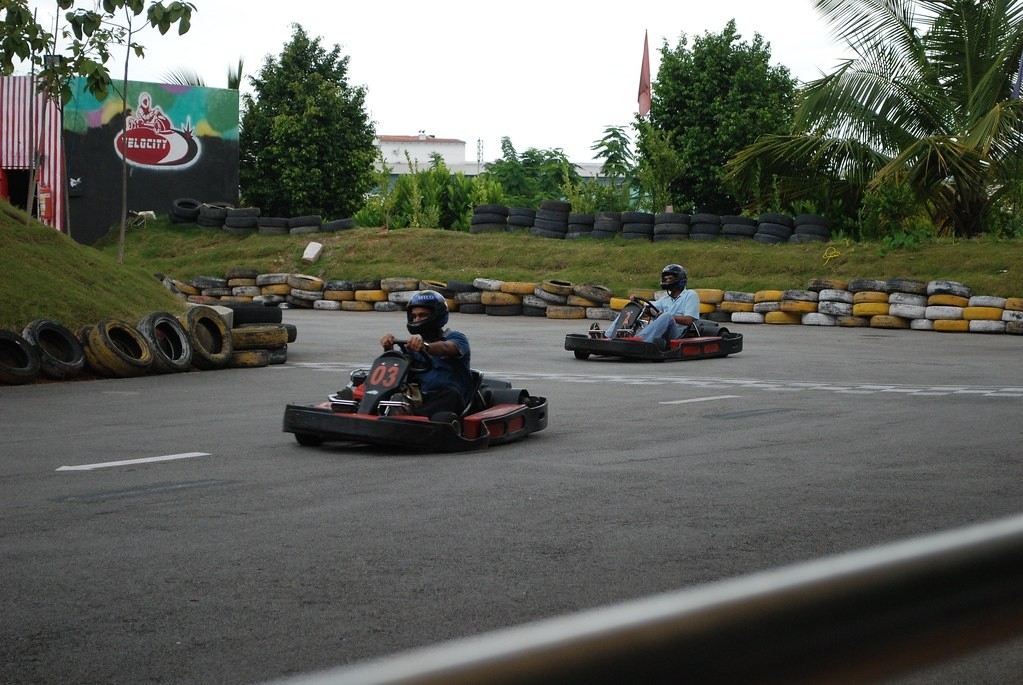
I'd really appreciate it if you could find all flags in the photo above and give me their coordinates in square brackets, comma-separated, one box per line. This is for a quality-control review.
[638, 30, 651, 118]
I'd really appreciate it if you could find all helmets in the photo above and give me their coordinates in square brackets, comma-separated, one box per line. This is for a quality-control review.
[660, 264, 687, 291]
[406, 290, 449, 335]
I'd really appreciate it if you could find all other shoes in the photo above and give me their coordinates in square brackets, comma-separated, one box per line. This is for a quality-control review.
[588, 323, 601, 338]
[389, 393, 408, 416]
[340, 389, 354, 412]
[619, 325, 631, 337]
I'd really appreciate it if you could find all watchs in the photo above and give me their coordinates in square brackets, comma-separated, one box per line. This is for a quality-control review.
[423, 340, 429, 352]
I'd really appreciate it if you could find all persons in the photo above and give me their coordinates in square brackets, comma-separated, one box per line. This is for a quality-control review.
[380, 290, 471, 416]
[604, 264, 699, 342]
